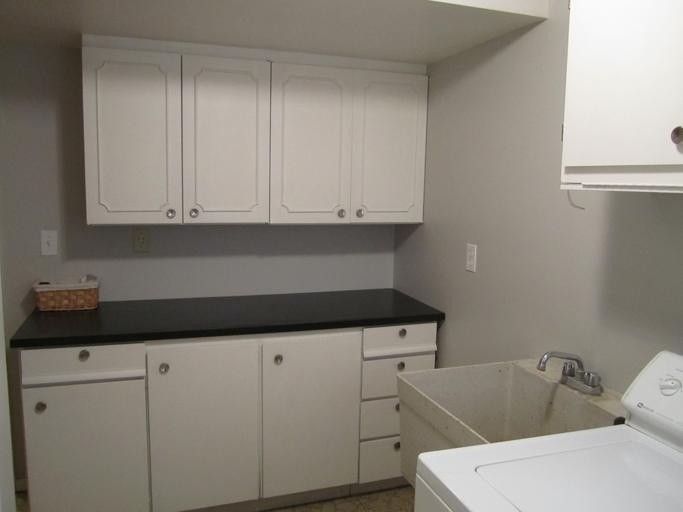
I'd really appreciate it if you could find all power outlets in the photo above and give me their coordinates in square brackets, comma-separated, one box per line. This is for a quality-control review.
[133, 229, 149, 253]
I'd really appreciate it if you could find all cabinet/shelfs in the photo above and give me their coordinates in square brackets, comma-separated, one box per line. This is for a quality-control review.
[270, 50, 429, 224]
[262, 325, 362, 511]
[17, 340, 151, 512]
[560, 1, 683, 193]
[361, 321, 437, 495]
[82, 45, 271, 226]
[146, 333, 262, 512]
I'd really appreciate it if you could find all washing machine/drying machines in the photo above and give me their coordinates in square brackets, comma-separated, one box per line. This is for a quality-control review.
[414, 351, 682, 512]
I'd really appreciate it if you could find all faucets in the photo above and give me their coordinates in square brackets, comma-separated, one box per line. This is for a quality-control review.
[535, 350, 604, 397]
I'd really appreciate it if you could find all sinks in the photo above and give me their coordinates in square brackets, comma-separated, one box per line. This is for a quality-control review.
[396, 355, 628, 446]
